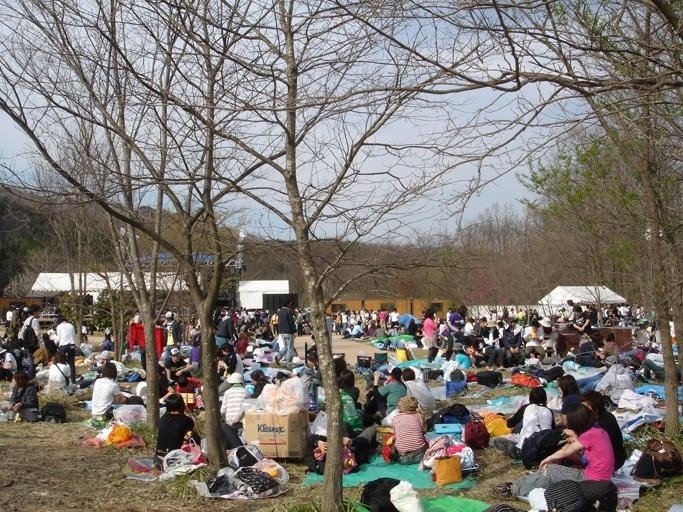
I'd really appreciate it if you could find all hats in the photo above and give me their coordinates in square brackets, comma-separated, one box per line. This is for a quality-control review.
[487, 320, 498, 328]
[544, 480, 586, 512]
[601, 355, 618, 366]
[398, 397, 417, 414]
[537, 317, 552, 328]
[0, 346, 6, 356]
[384, 367, 402, 377]
[169, 347, 180, 356]
[562, 361, 578, 375]
[164, 312, 175, 319]
[226, 373, 244, 384]
[452, 342, 463, 351]
[227, 309, 235, 316]
[94, 350, 111, 360]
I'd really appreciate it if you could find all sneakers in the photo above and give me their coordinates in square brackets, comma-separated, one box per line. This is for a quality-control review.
[488, 489, 514, 501]
[274, 356, 280, 367]
[494, 438, 519, 459]
[490, 481, 514, 493]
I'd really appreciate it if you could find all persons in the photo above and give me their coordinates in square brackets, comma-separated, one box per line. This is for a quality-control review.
[1, 295, 681, 512]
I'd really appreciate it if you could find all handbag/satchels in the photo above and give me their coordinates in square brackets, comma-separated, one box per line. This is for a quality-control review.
[446, 405, 469, 420]
[512, 374, 539, 388]
[447, 439, 475, 469]
[434, 455, 463, 485]
[181, 438, 206, 465]
[418, 435, 448, 471]
[484, 413, 514, 436]
[618, 390, 658, 411]
[465, 412, 490, 449]
[631, 438, 682, 481]
[20, 408, 39, 423]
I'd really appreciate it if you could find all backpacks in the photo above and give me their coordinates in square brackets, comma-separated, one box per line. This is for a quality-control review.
[477, 371, 502, 388]
[119, 372, 141, 382]
[42, 402, 66, 423]
[360, 477, 399, 511]
[521, 428, 562, 471]
[23, 318, 37, 348]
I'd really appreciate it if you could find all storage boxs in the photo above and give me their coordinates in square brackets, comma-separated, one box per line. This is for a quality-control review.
[244, 411, 311, 461]
